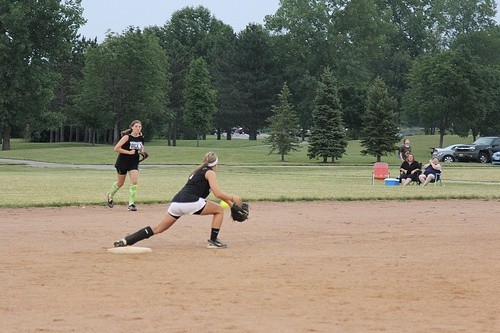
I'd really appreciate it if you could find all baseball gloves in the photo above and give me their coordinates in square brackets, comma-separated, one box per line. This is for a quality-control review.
[138, 151, 148, 162]
[231, 202, 249, 223]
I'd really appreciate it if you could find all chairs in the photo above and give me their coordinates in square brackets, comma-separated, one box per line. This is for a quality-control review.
[372, 161, 441, 186]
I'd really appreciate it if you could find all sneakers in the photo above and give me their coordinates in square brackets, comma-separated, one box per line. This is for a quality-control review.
[128, 204, 137, 211]
[114, 239, 127, 247]
[207, 239, 227, 248]
[107, 193, 113, 208]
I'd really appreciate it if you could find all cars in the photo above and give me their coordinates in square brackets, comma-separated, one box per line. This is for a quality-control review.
[289, 129, 312, 137]
[432, 143, 467, 163]
[215, 126, 262, 134]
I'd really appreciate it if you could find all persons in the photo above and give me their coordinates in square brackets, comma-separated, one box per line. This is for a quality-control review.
[419, 156, 442, 186]
[399, 138, 412, 162]
[475, 132, 481, 140]
[399, 154, 420, 187]
[107, 120, 145, 211]
[113, 152, 244, 249]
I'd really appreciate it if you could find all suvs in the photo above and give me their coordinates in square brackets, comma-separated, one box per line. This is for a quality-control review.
[451, 136, 500, 164]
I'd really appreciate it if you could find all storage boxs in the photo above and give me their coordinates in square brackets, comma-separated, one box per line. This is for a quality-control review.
[384, 178, 400, 186]
